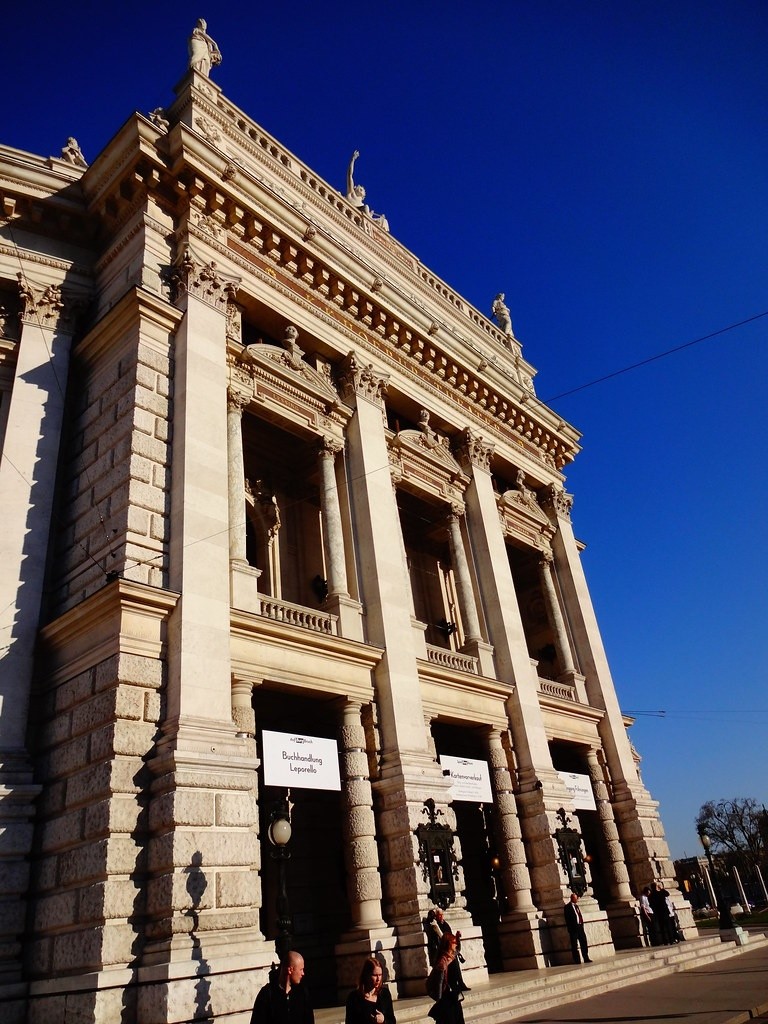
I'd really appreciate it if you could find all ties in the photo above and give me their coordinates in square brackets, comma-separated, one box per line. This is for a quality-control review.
[574, 905, 583, 927]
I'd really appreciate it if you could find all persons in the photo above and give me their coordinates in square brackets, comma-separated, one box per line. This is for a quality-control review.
[491, 291, 515, 337]
[416, 409, 431, 429]
[643, 882, 679, 947]
[564, 894, 595, 964]
[514, 469, 526, 486]
[345, 957, 398, 1023]
[250, 950, 315, 1024]
[282, 326, 299, 346]
[428, 933, 464, 1023]
[344, 147, 378, 218]
[426, 907, 471, 992]
[60, 136, 89, 167]
[187, 17, 222, 80]
[149, 107, 171, 135]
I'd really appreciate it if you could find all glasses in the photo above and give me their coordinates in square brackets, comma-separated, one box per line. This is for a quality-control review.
[453, 941, 458, 945]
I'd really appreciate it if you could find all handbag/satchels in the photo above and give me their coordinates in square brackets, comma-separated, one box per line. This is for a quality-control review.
[425, 956, 448, 1002]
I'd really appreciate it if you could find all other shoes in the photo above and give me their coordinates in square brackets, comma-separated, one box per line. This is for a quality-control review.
[584, 959, 593, 963]
[461, 987, 471, 990]
[575, 961, 581, 964]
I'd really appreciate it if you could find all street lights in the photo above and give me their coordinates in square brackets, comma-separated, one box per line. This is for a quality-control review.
[267, 800, 296, 963]
[696, 822, 743, 942]
